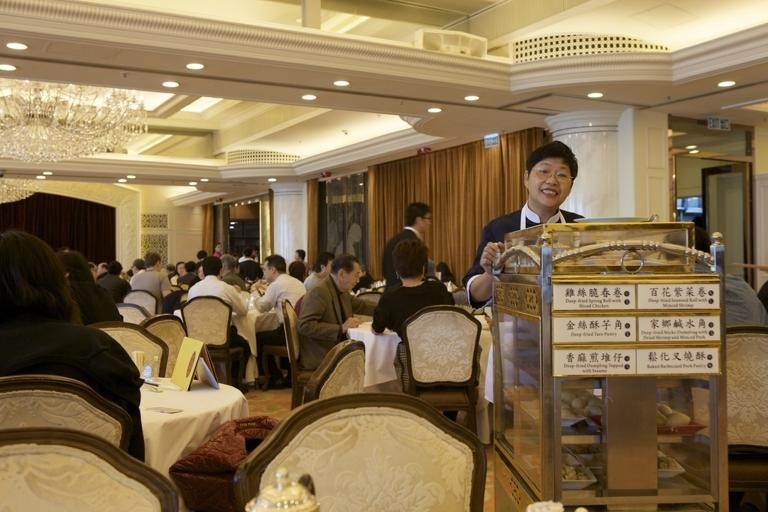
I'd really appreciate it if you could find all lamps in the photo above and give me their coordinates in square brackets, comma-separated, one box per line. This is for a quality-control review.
[0, 80, 148, 164]
[0, 178, 39, 205]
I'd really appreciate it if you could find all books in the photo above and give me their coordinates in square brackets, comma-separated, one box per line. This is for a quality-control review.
[171, 336, 221, 393]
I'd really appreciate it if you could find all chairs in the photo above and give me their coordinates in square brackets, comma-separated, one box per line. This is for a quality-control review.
[122, 289, 158, 318]
[139, 314, 188, 379]
[261, 344, 290, 391]
[355, 292, 383, 304]
[232, 392, 488, 512]
[400, 304, 483, 419]
[116, 303, 151, 323]
[181, 295, 247, 396]
[87, 320, 169, 378]
[281, 298, 317, 410]
[0, 426, 179, 511]
[726, 325, 768, 512]
[0, 374, 134, 454]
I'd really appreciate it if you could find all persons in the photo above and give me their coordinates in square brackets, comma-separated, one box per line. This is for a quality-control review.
[239, 247, 264, 282]
[96, 260, 132, 303]
[251, 254, 306, 389]
[127, 259, 146, 277]
[0, 229, 147, 463]
[188, 261, 206, 289]
[238, 246, 259, 264]
[461, 140, 585, 444]
[294, 249, 310, 277]
[381, 201, 432, 284]
[294, 253, 362, 371]
[371, 238, 468, 425]
[186, 256, 251, 379]
[303, 251, 336, 290]
[197, 250, 207, 262]
[656, 225, 767, 332]
[170, 261, 188, 286]
[56, 249, 124, 326]
[97, 262, 108, 276]
[130, 251, 172, 314]
[212, 241, 224, 257]
[219, 254, 246, 291]
[176, 260, 197, 286]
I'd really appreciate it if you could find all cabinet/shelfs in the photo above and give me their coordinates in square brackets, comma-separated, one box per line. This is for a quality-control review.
[488, 221, 728, 512]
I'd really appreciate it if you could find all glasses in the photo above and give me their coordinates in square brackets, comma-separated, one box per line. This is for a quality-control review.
[531, 166, 573, 184]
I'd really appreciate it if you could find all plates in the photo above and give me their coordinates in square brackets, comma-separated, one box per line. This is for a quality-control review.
[657, 420, 707, 435]
[522, 453, 580, 468]
[561, 468, 597, 492]
[579, 446, 686, 481]
